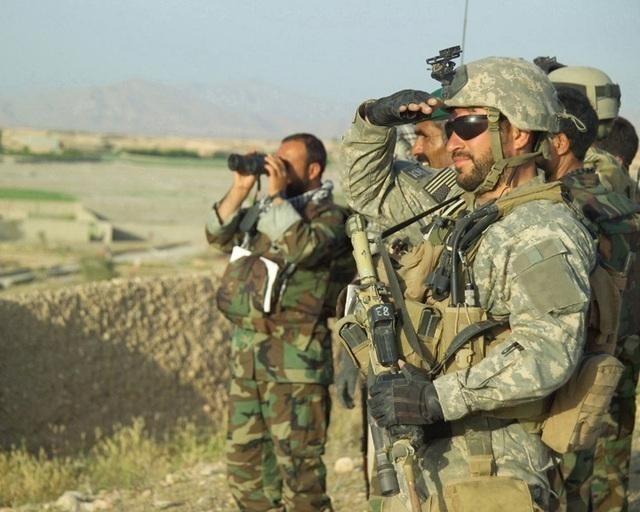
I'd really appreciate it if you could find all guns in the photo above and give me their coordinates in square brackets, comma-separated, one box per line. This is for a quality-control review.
[344, 215, 423, 512]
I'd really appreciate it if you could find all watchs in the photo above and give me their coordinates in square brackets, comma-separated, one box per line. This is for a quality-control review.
[268, 189, 288, 202]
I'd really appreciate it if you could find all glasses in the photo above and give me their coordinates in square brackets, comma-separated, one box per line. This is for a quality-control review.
[444, 114, 506, 141]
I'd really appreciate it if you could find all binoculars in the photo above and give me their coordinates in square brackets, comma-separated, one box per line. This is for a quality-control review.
[228, 153, 288, 175]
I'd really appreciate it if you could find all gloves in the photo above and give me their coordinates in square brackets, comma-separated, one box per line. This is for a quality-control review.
[335, 357, 358, 409]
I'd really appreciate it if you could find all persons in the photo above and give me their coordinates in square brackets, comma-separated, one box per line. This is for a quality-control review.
[324, 55, 640, 511]
[205, 132, 350, 512]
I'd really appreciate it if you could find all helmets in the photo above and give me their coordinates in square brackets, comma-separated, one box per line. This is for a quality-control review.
[441, 57, 561, 133]
[547, 66, 619, 120]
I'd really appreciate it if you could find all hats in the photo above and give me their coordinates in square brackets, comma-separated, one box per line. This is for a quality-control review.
[413, 88, 449, 124]
